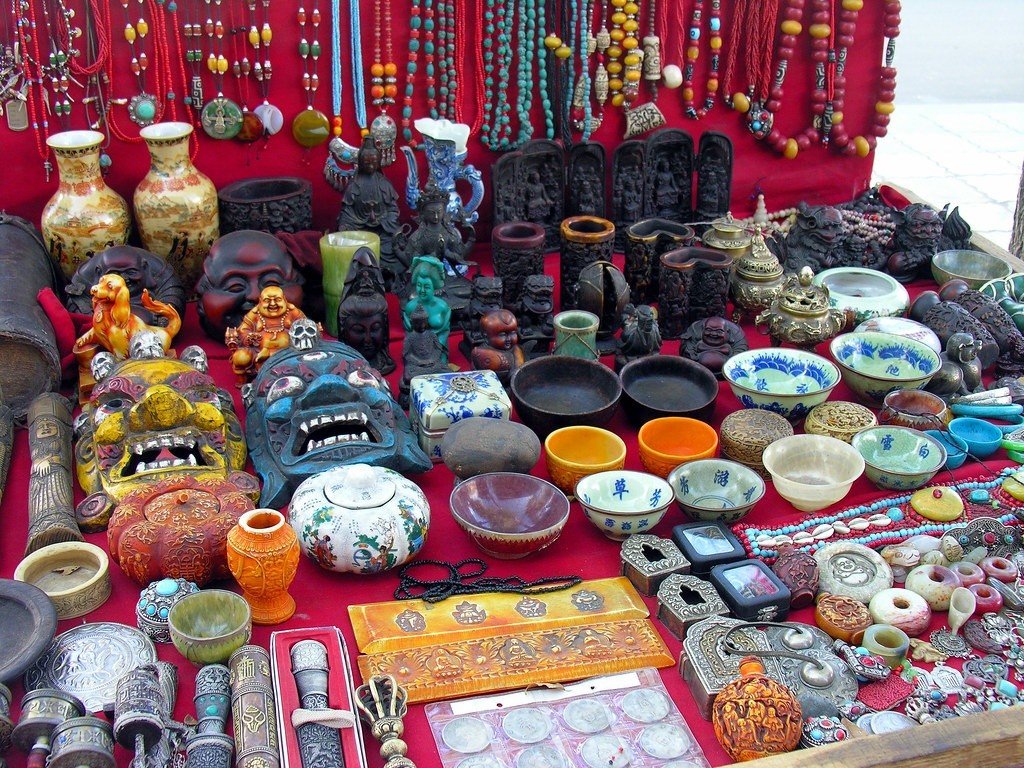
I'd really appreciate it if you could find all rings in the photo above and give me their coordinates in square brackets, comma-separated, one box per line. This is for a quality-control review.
[841, 702, 865, 721]
[931, 690, 948, 703]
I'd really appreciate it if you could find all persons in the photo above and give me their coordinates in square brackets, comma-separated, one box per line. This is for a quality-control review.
[526, 152, 724, 222]
[680, 317, 748, 374]
[335, 248, 389, 376]
[359, 270, 374, 293]
[195, 229, 307, 345]
[408, 186, 476, 318]
[66, 245, 187, 343]
[226, 286, 322, 389]
[337, 134, 400, 269]
[619, 304, 663, 354]
[401, 256, 453, 380]
[471, 308, 526, 372]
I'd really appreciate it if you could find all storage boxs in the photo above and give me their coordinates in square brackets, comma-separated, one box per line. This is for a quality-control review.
[710, 559, 791, 623]
[672, 520, 747, 581]
[408, 368, 512, 462]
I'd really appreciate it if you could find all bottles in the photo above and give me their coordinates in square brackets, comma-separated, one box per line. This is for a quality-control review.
[40, 129, 134, 285]
[224, 508, 302, 625]
[132, 121, 220, 303]
[908, 272, 1024, 368]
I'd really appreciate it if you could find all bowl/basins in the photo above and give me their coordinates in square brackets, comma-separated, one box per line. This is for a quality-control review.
[761, 433, 866, 512]
[667, 457, 767, 524]
[721, 346, 842, 427]
[545, 425, 626, 494]
[510, 354, 622, 441]
[827, 332, 943, 410]
[881, 389, 946, 432]
[573, 470, 676, 542]
[168, 589, 253, 668]
[852, 425, 948, 490]
[931, 249, 1013, 290]
[619, 354, 719, 428]
[948, 417, 1003, 458]
[638, 415, 718, 476]
[449, 471, 571, 560]
[921, 429, 969, 471]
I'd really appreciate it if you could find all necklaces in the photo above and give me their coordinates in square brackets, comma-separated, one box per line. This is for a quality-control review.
[257, 308, 289, 340]
[0, 0, 901, 184]
[393, 558, 582, 604]
[743, 195, 898, 245]
[733, 462, 1024, 570]
